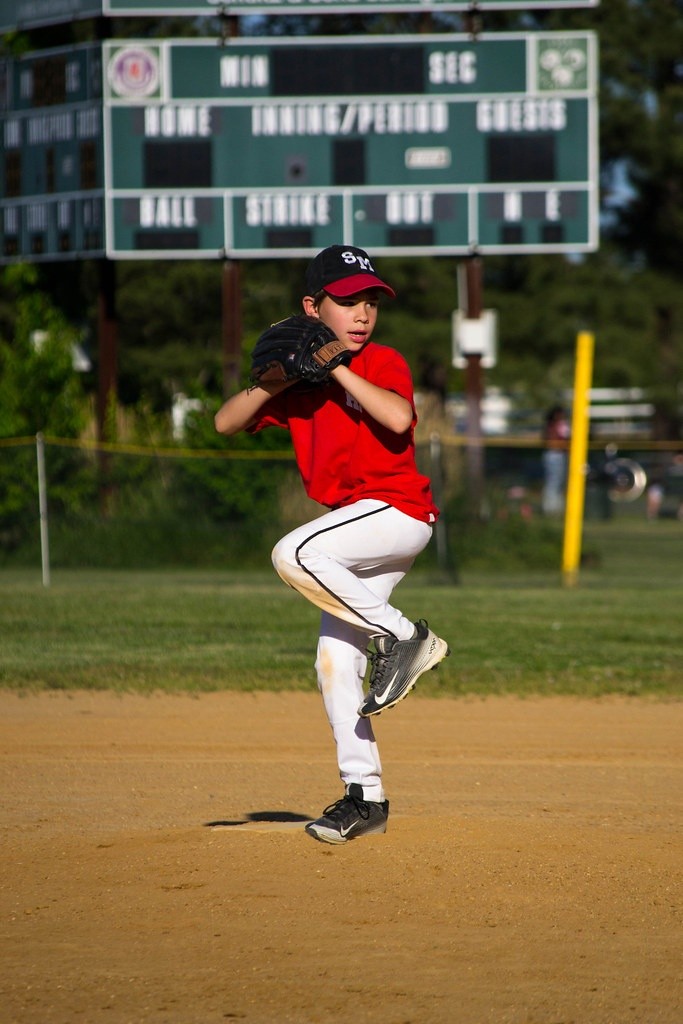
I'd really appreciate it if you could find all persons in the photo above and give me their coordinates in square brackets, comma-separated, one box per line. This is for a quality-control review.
[541, 403, 571, 516]
[214, 244, 451, 845]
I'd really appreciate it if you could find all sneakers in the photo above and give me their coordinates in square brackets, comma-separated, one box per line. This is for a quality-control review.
[357, 619, 450, 719]
[305, 783, 389, 844]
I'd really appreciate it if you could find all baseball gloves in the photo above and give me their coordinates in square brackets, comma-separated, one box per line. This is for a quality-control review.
[248, 314, 353, 388]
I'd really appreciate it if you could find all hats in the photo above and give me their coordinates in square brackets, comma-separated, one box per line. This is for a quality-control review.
[303, 245, 397, 299]
